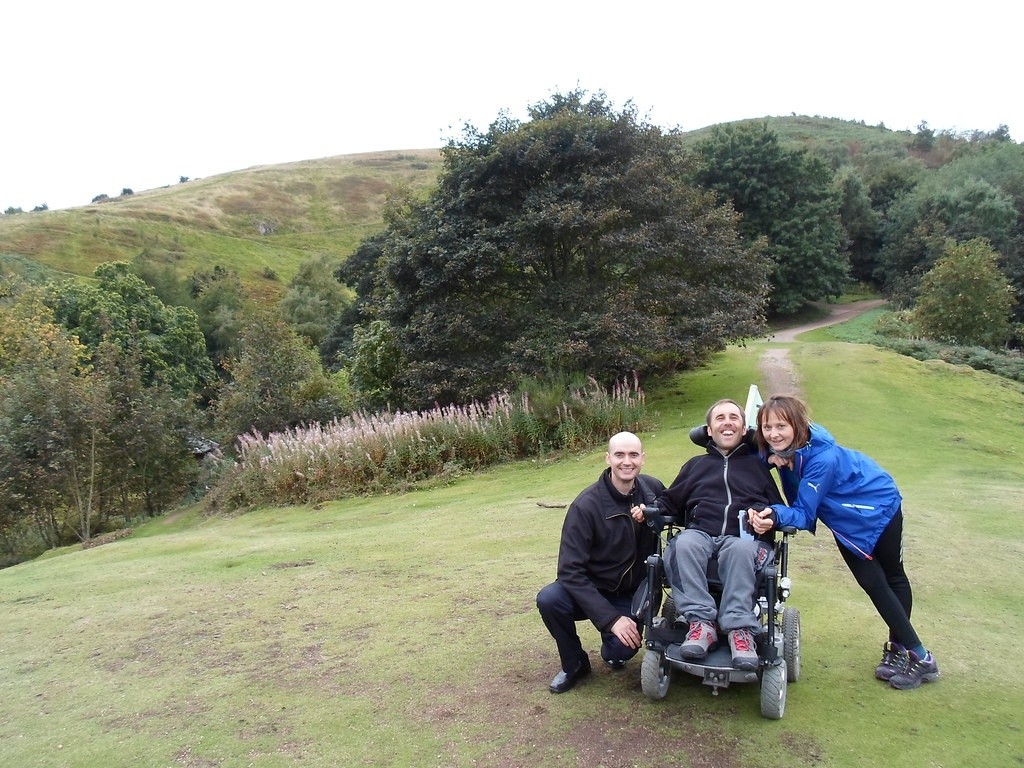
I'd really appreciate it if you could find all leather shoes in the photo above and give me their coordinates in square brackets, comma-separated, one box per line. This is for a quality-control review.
[605, 659, 628, 668]
[549, 652, 591, 693]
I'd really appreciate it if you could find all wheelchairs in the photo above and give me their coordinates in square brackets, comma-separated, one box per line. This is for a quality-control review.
[628, 423, 803, 720]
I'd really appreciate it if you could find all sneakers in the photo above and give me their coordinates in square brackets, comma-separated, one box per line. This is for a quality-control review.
[679, 621, 719, 659]
[728, 627, 759, 670]
[874, 641, 906, 680]
[889, 650, 940, 690]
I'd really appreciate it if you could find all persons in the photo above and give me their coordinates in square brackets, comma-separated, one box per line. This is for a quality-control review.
[753, 395, 939, 688]
[631, 398, 782, 672]
[537, 432, 666, 693]
